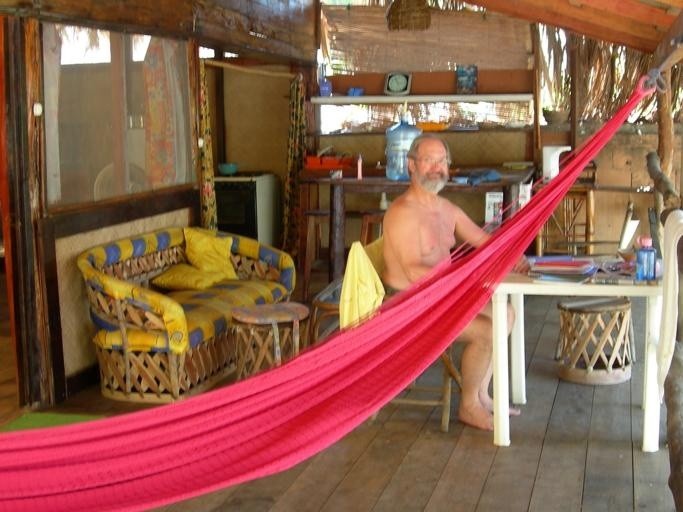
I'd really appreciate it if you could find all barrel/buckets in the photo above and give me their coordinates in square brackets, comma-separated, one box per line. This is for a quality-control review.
[385, 113, 422, 181]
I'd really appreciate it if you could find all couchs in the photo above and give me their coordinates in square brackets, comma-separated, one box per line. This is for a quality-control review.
[75, 223, 298, 404]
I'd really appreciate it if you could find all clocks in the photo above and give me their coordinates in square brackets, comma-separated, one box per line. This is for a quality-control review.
[383, 72, 413, 95]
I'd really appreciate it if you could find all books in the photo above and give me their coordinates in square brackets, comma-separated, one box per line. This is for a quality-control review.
[529, 261, 597, 284]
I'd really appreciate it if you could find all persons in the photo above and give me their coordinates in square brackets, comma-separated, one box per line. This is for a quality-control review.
[381, 132, 532, 430]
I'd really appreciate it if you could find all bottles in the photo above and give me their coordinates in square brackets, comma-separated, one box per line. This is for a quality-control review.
[320, 77, 333, 97]
[357, 153, 363, 180]
[634, 235, 657, 282]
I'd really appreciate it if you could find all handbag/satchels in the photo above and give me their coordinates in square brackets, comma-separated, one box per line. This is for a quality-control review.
[452, 168, 502, 187]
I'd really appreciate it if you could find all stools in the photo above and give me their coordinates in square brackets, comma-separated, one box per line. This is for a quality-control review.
[558, 296, 635, 385]
[231, 300, 311, 384]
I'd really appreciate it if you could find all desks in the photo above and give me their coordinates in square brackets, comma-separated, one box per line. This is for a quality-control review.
[486, 255, 664, 453]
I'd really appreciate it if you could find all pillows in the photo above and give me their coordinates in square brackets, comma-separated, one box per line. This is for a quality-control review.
[182, 226, 240, 283]
[151, 262, 213, 289]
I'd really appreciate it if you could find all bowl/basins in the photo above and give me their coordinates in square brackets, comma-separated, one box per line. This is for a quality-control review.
[542, 106, 571, 126]
[217, 162, 238, 176]
[617, 248, 637, 262]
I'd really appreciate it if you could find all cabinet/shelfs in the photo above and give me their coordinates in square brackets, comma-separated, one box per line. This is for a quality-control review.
[295, 67, 544, 295]
[533, 180, 595, 257]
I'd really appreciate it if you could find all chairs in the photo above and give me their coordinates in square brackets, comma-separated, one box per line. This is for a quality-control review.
[338, 235, 462, 432]
[309, 210, 383, 346]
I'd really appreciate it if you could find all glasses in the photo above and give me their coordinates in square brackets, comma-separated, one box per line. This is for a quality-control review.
[409, 154, 452, 168]
